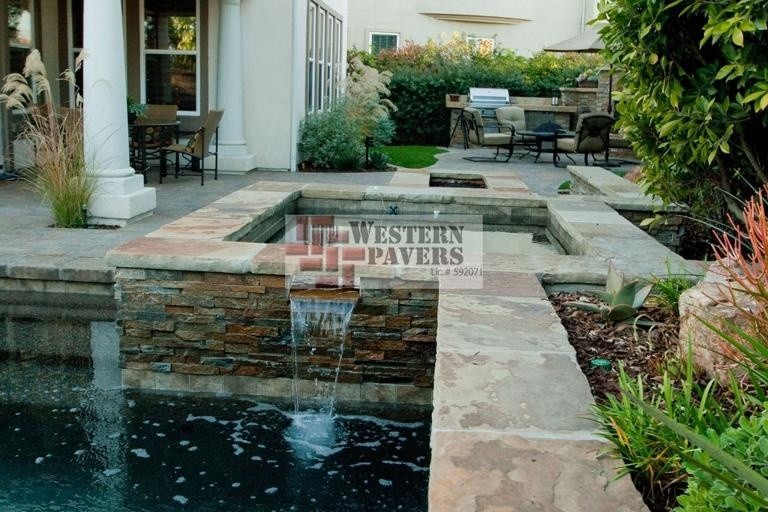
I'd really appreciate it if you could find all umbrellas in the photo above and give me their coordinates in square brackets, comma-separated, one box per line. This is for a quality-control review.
[542, 23, 619, 114]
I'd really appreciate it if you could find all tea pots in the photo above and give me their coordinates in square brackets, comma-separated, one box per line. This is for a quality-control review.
[551, 90, 559, 106]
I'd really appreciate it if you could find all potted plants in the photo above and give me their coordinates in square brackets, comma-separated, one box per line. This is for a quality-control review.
[128, 94, 140, 124]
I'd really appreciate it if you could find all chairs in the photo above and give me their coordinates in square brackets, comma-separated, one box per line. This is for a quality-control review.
[162, 108, 225, 188]
[553, 111, 616, 169]
[495, 106, 536, 160]
[458, 106, 515, 164]
[134, 104, 177, 178]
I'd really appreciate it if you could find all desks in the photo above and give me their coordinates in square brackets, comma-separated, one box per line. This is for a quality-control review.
[515, 132, 579, 166]
[128, 123, 181, 185]
